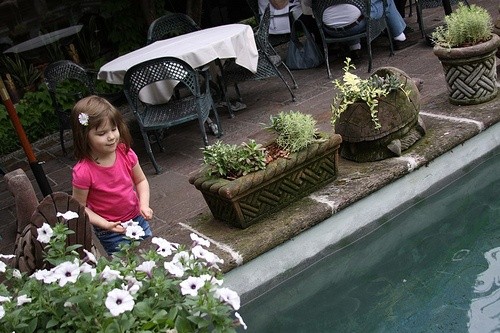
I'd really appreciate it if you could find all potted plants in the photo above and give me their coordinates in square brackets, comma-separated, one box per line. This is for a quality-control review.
[330, 57, 426, 163]
[428, 2, 500, 105]
[188, 109, 343, 229]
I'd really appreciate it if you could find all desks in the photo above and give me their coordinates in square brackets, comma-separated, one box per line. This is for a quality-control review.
[96, 23, 259, 153]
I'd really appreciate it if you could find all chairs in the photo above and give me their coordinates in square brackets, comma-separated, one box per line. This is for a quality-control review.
[46, 0, 472, 176]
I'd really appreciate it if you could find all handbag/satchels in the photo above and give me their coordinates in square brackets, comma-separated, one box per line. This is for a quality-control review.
[285, 18, 324, 70]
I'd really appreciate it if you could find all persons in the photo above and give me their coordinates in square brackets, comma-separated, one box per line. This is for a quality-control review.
[258, 0, 337, 61]
[321, 0, 418, 59]
[71, 95, 154, 255]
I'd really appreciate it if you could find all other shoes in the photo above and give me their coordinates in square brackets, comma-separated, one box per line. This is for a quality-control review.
[349, 46, 367, 63]
[393, 36, 417, 50]
[403, 25, 415, 32]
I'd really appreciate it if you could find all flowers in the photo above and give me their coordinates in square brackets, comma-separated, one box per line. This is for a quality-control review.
[0, 210, 248, 333]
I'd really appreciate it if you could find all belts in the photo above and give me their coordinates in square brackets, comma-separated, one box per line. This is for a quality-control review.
[321, 14, 362, 31]
